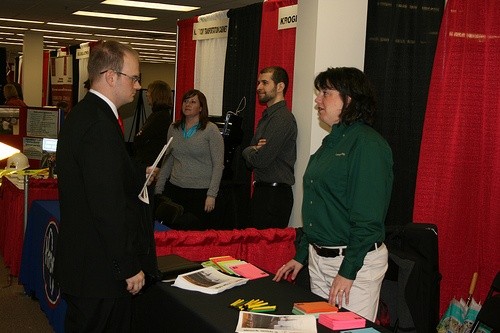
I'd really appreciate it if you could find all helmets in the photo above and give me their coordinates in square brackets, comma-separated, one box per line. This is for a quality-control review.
[6, 152, 31, 171]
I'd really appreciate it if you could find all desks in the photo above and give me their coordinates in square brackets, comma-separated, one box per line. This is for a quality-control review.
[126, 258, 394, 333]
[0, 134, 178, 333]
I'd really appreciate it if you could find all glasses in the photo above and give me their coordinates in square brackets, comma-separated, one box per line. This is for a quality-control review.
[100, 69, 142, 85]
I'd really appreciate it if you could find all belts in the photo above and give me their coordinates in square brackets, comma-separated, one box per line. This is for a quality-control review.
[311, 240, 383, 259]
[250, 180, 288, 188]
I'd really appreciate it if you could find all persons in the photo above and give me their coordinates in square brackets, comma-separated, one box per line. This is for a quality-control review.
[273, 68, 394, 323]
[3, 84, 27, 106]
[56, 39, 224, 333]
[242, 66, 298, 227]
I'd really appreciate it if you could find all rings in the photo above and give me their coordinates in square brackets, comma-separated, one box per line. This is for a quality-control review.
[338, 289, 344, 296]
[282, 264, 286, 268]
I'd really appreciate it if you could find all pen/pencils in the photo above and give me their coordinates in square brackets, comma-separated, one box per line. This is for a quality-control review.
[230, 298, 276, 315]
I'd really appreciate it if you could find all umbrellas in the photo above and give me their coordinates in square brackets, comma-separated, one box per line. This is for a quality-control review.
[436, 272, 482, 333]
